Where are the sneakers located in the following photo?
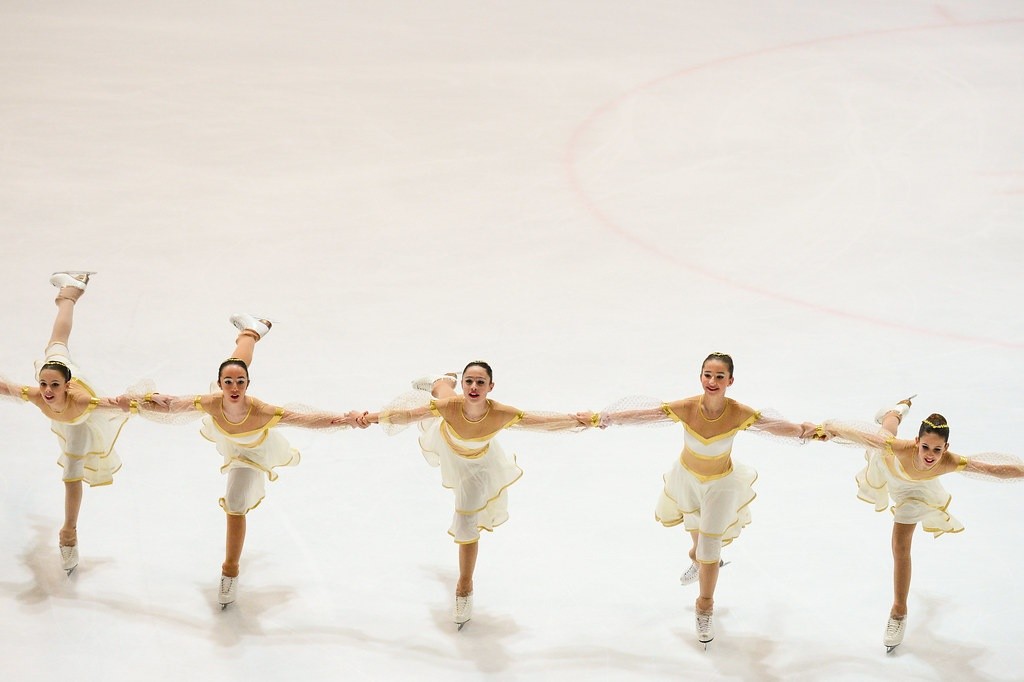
[229,312,272,345]
[453,579,473,631]
[883,605,908,653]
[695,595,716,650]
[680,548,731,585]
[49,270,97,305]
[60,526,79,576]
[412,371,462,395]
[217,563,240,611]
[875,394,917,425]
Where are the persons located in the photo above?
[1,270,1024,653]
